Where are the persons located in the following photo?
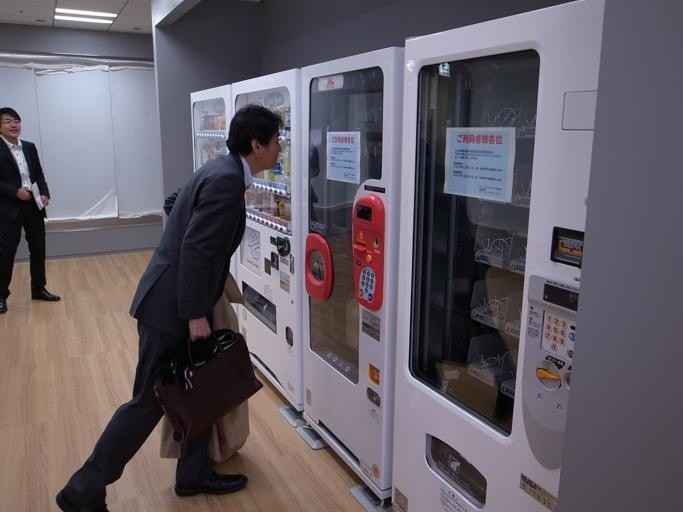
[50,103,284,511]
[0,106,61,314]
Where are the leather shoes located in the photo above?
[174,470,248,498]
[32,289,61,302]
[55,485,111,512]
[0,296,7,314]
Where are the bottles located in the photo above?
[247,103,290,220]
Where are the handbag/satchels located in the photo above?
[152,327,266,444]
